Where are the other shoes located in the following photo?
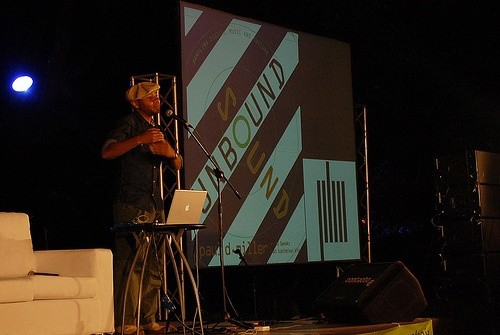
[142,323,163,331]
[115,324,137,335]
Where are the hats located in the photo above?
[125,81,160,100]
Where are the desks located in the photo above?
[109,223,208,335]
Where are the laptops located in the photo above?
[156,189,207,225]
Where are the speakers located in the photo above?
[317,261,428,326]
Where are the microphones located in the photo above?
[166,110,192,128]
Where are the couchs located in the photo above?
[0,212,115,335]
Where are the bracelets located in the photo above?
[170,153,178,159]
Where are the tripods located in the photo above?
[177,123,254,331]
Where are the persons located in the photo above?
[101,81,183,335]
